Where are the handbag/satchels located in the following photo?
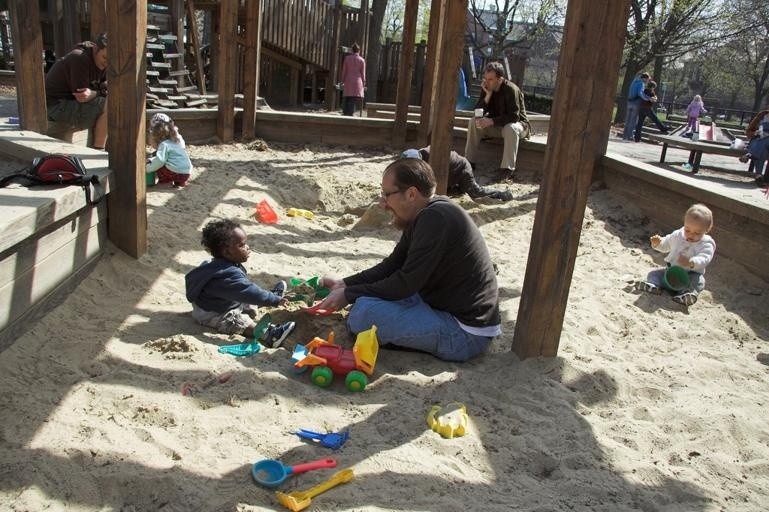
[28,153,93,186]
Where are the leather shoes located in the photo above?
[500,173,514,185]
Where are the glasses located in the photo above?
[381,189,407,199]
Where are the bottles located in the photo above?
[732,138,735,148]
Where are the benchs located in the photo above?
[648,132,755,173]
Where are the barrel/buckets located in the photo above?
[663,265,690,292]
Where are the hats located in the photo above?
[150,113,174,132]
[401,149,422,161]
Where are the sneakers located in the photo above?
[739,155,748,163]
[673,294,697,306]
[271,280,287,297]
[153,181,174,189]
[627,137,635,141]
[268,321,295,349]
[634,281,662,295]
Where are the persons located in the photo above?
[146,112,194,187]
[634,201,718,307]
[43,32,108,151]
[622,71,769,188]
[399,144,515,201]
[340,41,367,116]
[462,61,533,185]
[184,219,296,349]
[318,155,503,364]
[44,49,57,74]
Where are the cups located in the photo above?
[474,108,484,120]
